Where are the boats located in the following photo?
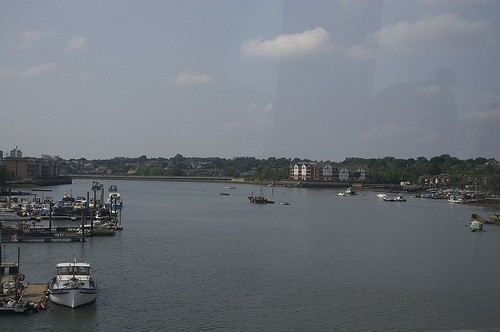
[469,213,484,232]
[279,201,290,205]
[224,186,237,190]
[447,194,463,203]
[376,193,387,198]
[0,242,26,301]
[382,193,406,203]
[337,186,358,197]
[0,181,123,220]
[46,254,98,309]
[220,192,230,196]
[248,185,276,204]
